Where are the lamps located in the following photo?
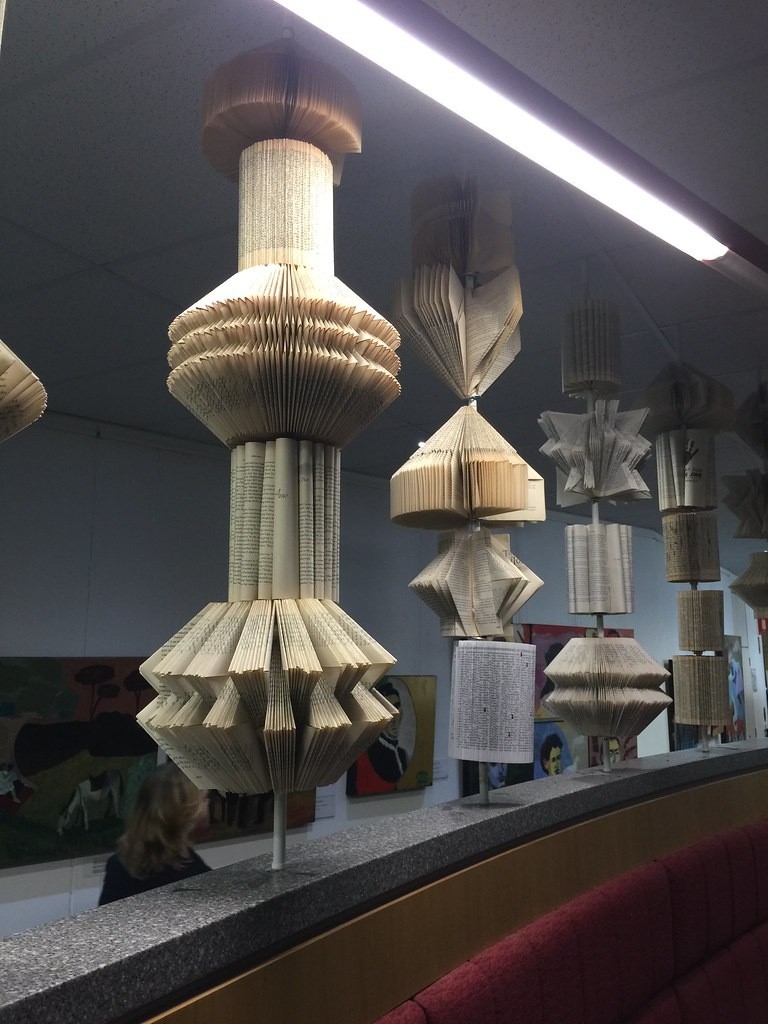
[275,1,768,299]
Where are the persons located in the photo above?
[98,759,214,906]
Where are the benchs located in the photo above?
[376,818,767,1024]
